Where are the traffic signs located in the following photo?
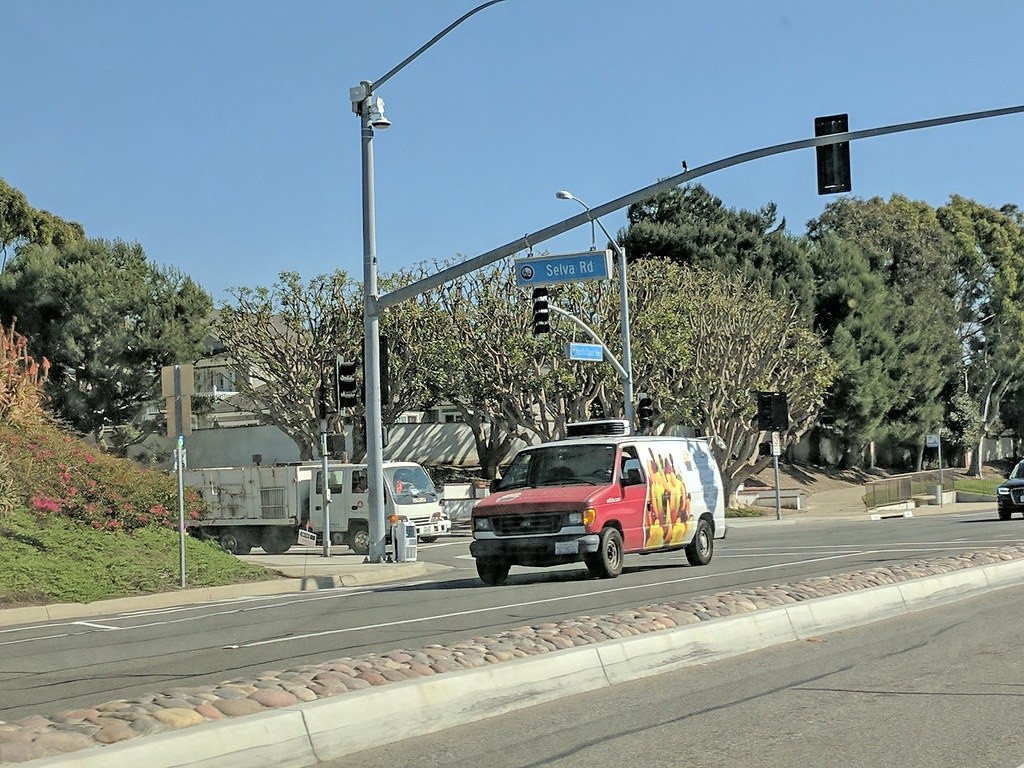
[514,250,612,287]
[564,342,603,362]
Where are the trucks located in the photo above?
[172,461,451,557]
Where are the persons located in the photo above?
[355,477,366,492]
[605,451,628,480]
[394,472,414,493]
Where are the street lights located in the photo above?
[554,191,634,435]
[360,97,393,559]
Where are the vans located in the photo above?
[471,420,726,586]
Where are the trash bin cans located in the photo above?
[392,521,417,562]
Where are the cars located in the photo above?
[997,460,1024,520]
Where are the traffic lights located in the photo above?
[757,392,774,431]
[774,392,789,431]
[335,356,357,415]
[530,286,550,335]
